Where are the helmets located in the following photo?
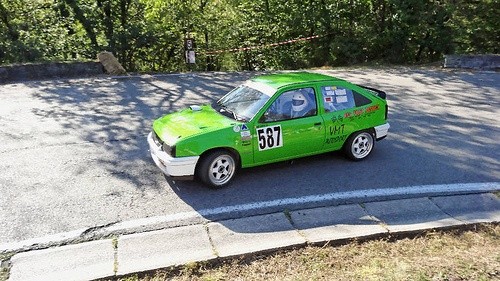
[292,91,310,111]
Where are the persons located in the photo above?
[286,93,313,122]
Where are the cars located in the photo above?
[147,71,391,188]
